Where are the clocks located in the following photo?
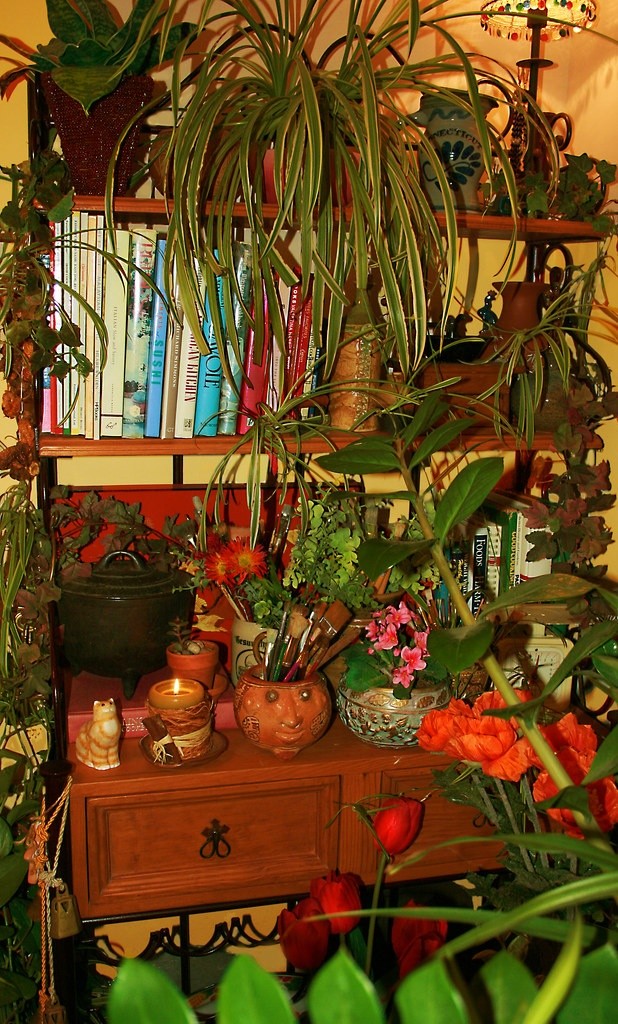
[494,638,573,714]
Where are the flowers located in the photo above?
[338,602,431,701]
[107,690,618,1024]
[48,476,448,630]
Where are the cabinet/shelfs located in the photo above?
[575,485,613,488]
[26,68,617,1024]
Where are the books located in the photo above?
[41,208,325,438]
[417,493,572,692]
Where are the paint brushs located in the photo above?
[263,599,352,683]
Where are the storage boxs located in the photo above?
[422,364,515,425]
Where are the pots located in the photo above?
[59,550,198,699]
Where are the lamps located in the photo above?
[479,0,597,181]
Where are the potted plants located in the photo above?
[164,615,219,688]
[104,0,561,383]
[0,0,205,198]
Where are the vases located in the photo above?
[233,616,281,688]
[149,127,262,200]
[338,676,453,749]
[418,95,499,214]
[327,281,388,432]
[481,282,551,362]
[511,351,584,431]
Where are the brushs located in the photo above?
[319,600,352,642]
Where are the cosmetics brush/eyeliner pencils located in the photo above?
[263,599,361,682]
